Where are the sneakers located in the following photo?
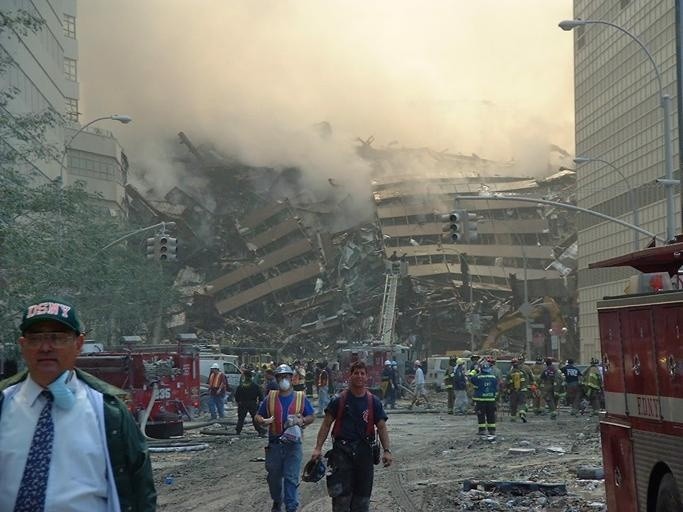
[271,500,281,512]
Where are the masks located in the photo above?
[279,379,290,391]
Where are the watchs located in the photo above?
[384,448,391,454]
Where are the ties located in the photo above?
[14,390,53,511]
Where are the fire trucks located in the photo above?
[72,343,202,437]
[337,272,408,399]
[586,240,682,512]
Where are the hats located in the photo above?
[20,297,80,335]
[415,360,422,367]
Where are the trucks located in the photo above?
[200,359,244,393]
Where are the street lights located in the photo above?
[55,114,132,259]
[559,20,683,247]
[575,158,641,250]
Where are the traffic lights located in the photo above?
[145,234,178,265]
[441,207,483,244]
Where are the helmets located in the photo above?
[275,364,293,375]
[568,359,574,364]
[511,353,524,364]
[302,456,325,482]
[384,360,397,366]
[536,355,552,365]
[210,363,219,369]
[483,355,495,369]
[592,359,599,365]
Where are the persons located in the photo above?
[409,359,431,409]
[444,354,604,422]
[255,363,315,512]
[208,353,400,433]
[467,359,497,435]
[311,361,393,512]
[0,298,158,512]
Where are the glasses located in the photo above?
[27,332,72,346]
